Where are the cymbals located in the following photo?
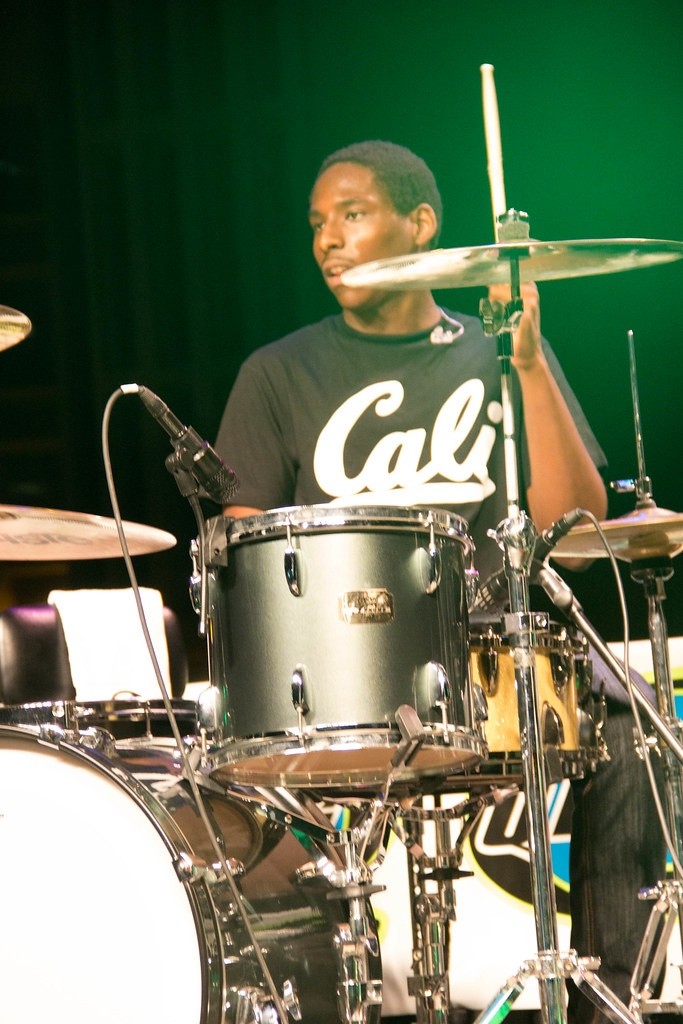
[547,501,683,562]
[0,303,33,353]
[340,237,683,289]
[0,503,179,562]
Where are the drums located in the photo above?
[467,609,606,779]
[193,504,490,790]
[0,724,389,1024]
[0,698,198,742]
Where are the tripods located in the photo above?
[474,512,683,1024]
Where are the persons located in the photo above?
[197,140,683,1023]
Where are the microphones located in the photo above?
[477,507,582,610]
[137,386,241,505]
